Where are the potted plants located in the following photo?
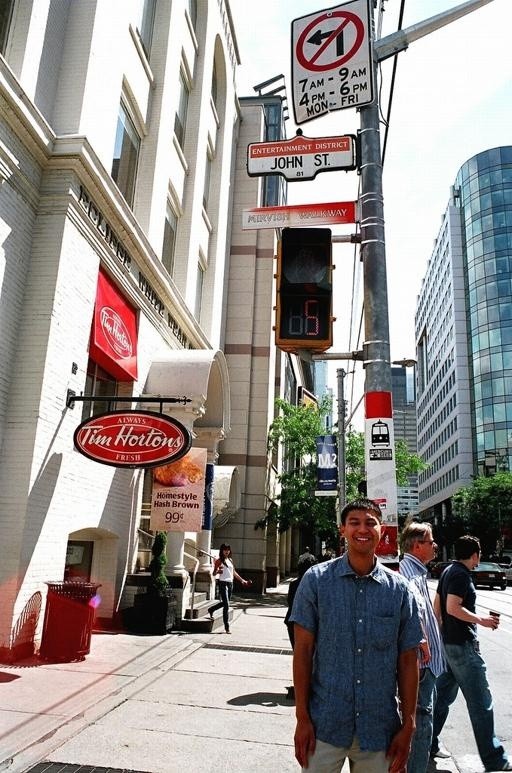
[132,531,178,635]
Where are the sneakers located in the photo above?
[208,608,214,619]
[502,755,512,770]
[226,630,231,633]
[435,749,452,758]
[286,687,295,700]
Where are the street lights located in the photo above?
[336,358,416,552]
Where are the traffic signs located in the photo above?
[246,135,354,182]
[241,200,357,230]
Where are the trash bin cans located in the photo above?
[39,581,103,663]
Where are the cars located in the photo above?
[427,560,512,590]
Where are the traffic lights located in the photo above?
[274,227,336,354]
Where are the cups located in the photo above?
[490,611,500,629]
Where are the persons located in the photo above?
[433,533,512,772]
[280,552,316,699]
[289,496,422,772]
[394,519,449,773]
[208,543,251,633]
